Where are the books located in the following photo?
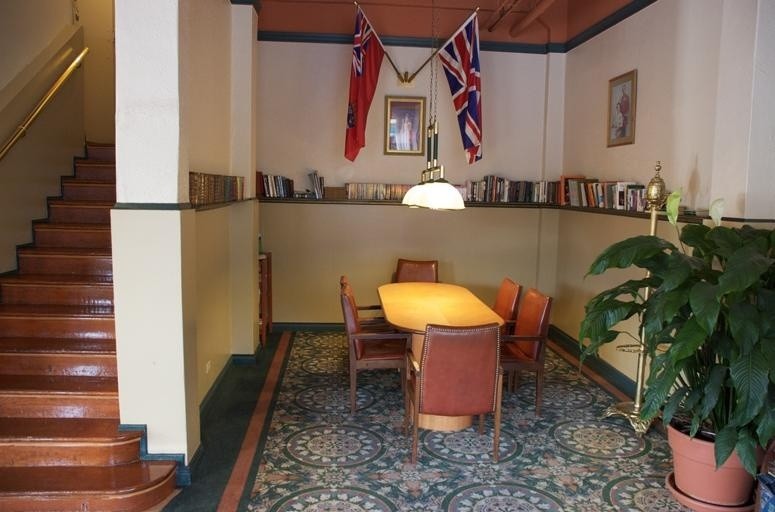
[293,192,316,199]
[561,175,667,213]
[453,176,561,205]
[678,206,696,215]
[345,183,415,200]
[256,171,294,198]
[308,172,323,199]
[320,177,324,195]
[190,172,244,207]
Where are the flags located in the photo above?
[342,7,384,163]
[437,12,483,166]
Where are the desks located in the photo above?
[376,282,504,432]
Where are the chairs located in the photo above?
[394,259,439,282]
[481,276,524,334]
[488,288,554,417]
[404,325,515,468]
[340,275,408,414]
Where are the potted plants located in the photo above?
[576,191,775,512]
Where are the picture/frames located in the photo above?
[383,95,427,156]
[607,68,637,147]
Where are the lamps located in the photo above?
[401,0,466,211]
